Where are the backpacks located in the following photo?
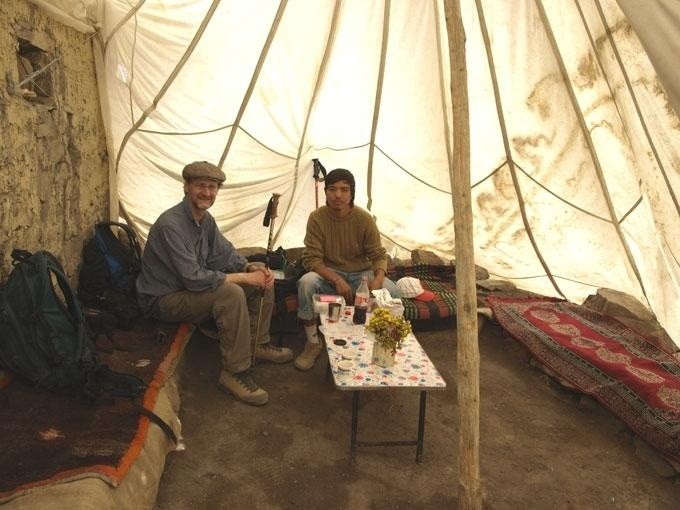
[0,248,178,448]
[77,219,143,308]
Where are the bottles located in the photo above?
[352,276,369,325]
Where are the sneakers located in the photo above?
[295,339,324,371]
[215,367,269,406]
[250,341,295,365]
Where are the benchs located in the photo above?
[267,264,480,328]
[0,311,198,509]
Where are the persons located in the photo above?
[135,161,294,405]
[294,168,402,372]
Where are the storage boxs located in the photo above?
[313,293,346,315]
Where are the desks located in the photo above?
[316,304,449,467]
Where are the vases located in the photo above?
[370,335,394,367]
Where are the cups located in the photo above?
[337,349,359,380]
[328,302,341,321]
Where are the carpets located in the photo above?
[486,296,680,460]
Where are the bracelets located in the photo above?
[333,276,342,284]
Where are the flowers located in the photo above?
[363,306,414,357]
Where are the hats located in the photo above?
[397,276,436,302]
[183,161,226,185]
